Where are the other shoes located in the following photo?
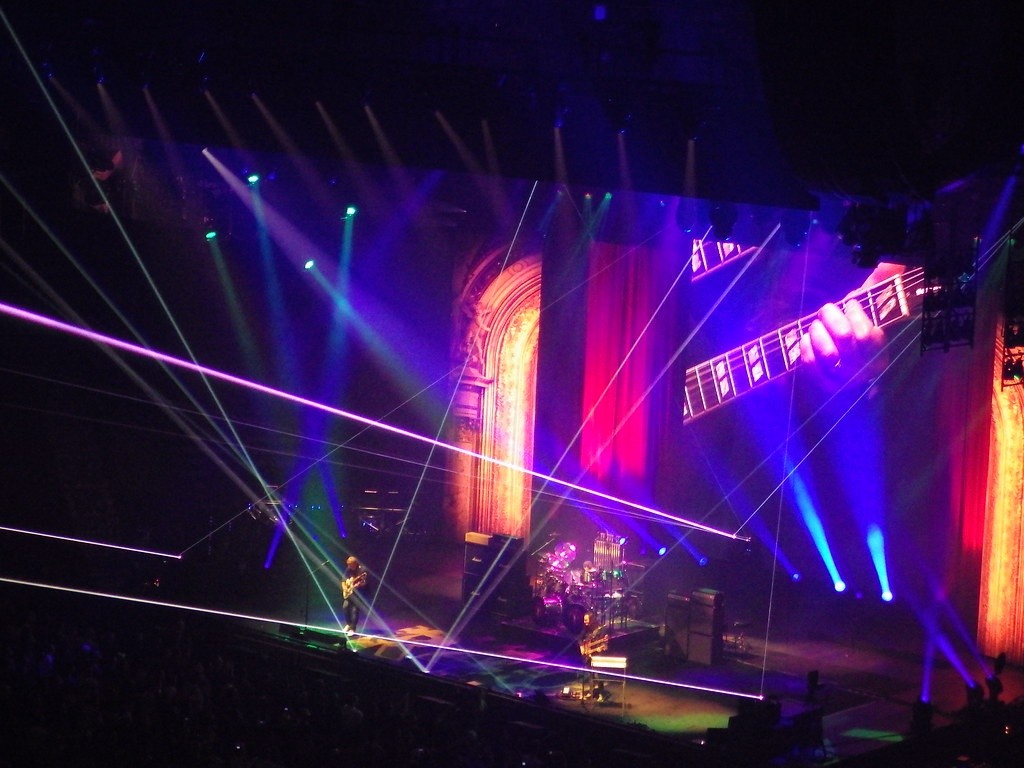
[347,630,355,637]
[342,624,351,634]
[597,690,609,702]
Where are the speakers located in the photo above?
[663,601,725,667]
[460,542,532,619]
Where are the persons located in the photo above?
[341,557,369,632]
[577,611,608,703]
[584,561,596,572]
[0,602,592,767]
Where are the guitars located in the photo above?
[340,571,368,600]
[682,261,932,432]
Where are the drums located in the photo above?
[562,600,598,635]
[532,592,564,627]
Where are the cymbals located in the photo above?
[555,542,577,563]
[546,553,569,569]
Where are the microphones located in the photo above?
[321,560,330,566]
[550,533,561,537]
